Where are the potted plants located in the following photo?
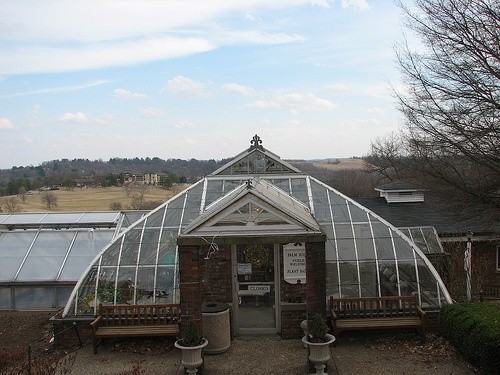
[174,317,208,369]
[302,312,337,375]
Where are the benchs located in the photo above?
[330,295,426,348]
[90,302,181,354]
